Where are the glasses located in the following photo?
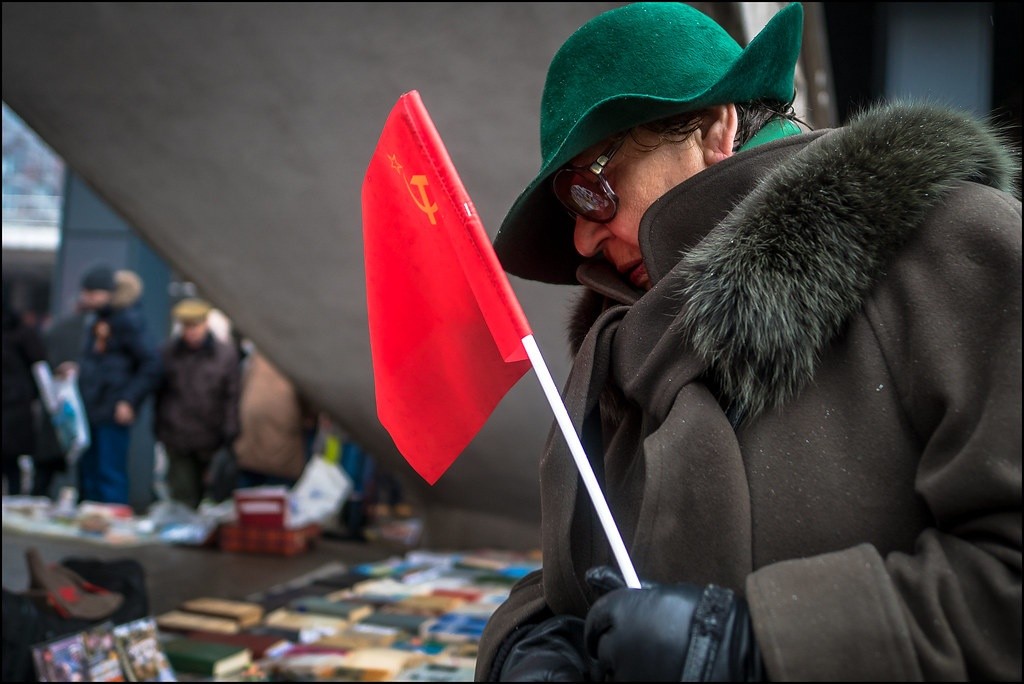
[553,111,649,221]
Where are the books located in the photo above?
[27,549,541,682]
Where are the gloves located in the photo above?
[581,566,763,684]
[501,615,587,684]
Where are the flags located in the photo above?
[358,88,539,488]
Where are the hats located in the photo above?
[175,297,207,323]
[493,1,805,286]
[83,265,117,292]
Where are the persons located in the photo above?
[0,270,53,496]
[140,295,240,504]
[240,337,307,488]
[472,1,1024,684]
[56,262,155,504]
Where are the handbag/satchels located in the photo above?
[46,369,92,465]
